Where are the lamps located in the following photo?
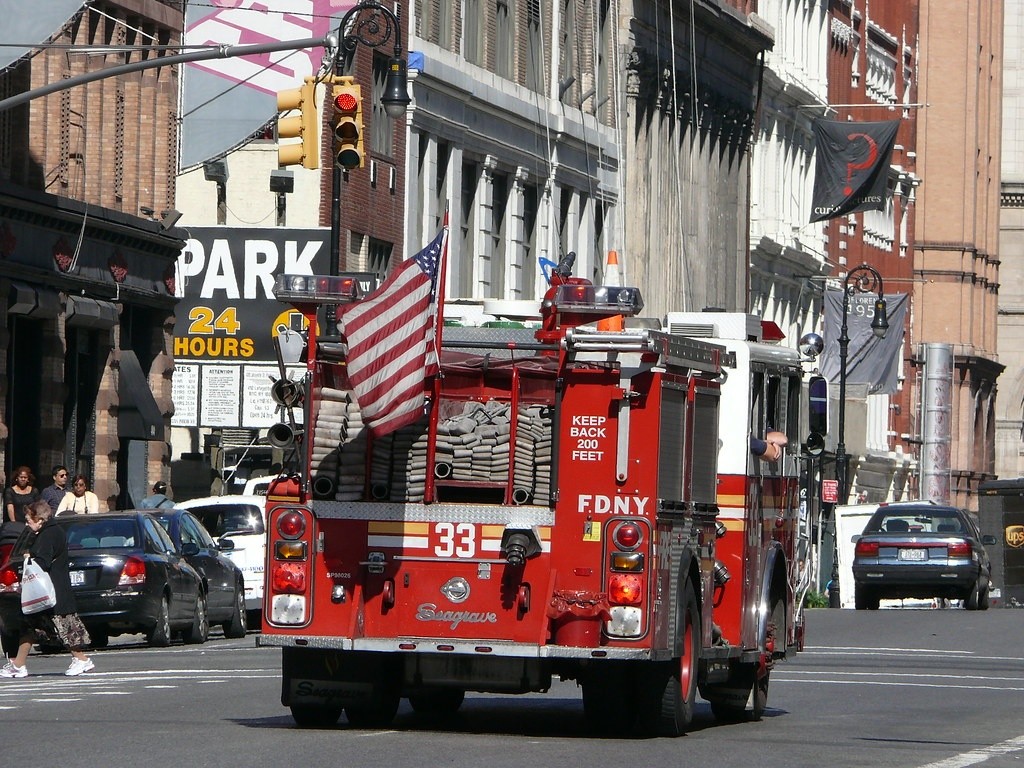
[162,208,183,231]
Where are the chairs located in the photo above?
[99,534,127,548]
[937,524,956,532]
[78,535,100,548]
[886,519,909,531]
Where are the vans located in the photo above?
[171,471,303,628]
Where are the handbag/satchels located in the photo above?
[21,555,56,613]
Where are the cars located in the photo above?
[849,502,993,611]
[7,509,248,647]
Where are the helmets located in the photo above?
[153,481,167,495]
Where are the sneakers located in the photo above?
[1,658,29,678]
[64,656,95,676]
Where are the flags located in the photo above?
[339,225,447,437]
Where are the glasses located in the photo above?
[57,472,69,478]
[73,481,86,487]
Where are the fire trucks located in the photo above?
[251,271,816,738]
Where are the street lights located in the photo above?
[833,265,890,508]
[323,1,412,335]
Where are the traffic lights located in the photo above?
[275,84,319,172]
[332,83,366,171]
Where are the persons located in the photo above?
[4,466,39,523]
[799,560,808,608]
[141,481,175,509]
[55,474,99,517]
[747,422,788,463]
[0,500,94,678]
[40,465,72,518]
